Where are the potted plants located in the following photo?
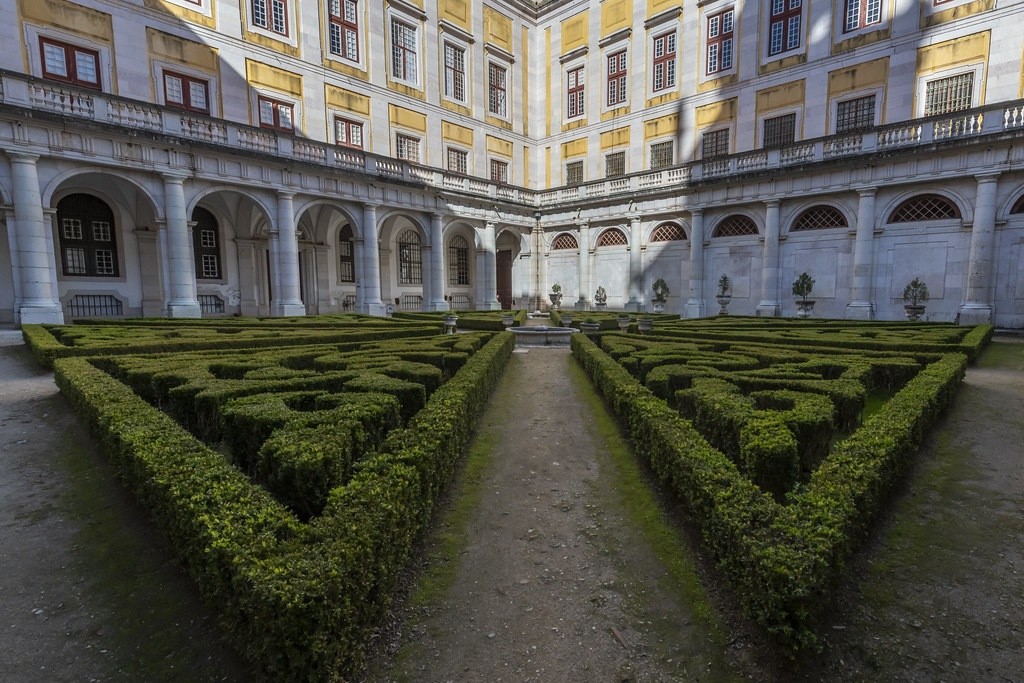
[548,283,563,311]
[650,278,669,313]
[716,274,733,315]
[791,272,819,319]
[901,277,929,320]
[594,286,608,311]
